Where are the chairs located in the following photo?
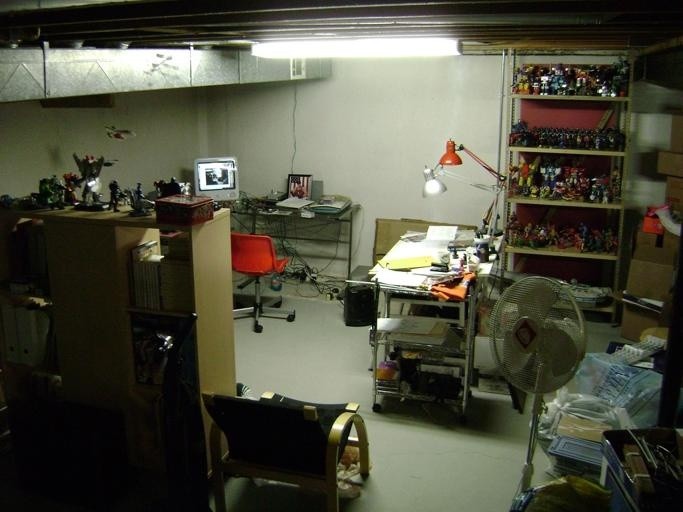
[198,388,375,511]
[228,232,297,334]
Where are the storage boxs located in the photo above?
[153,193,216,227]
[604,112,683,367]
[372,218,481,266]
[472,331,511,397]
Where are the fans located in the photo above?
[487,275,589,512]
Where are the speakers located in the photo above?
[344,286,374,326]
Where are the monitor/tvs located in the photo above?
[194,156,240,201]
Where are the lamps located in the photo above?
[421,164,502,262]
[247,36,468,62]
[438,134,508,238]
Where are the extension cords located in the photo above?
[285,273,318,284]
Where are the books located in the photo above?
[128,240,165,312]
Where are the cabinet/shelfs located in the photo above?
[501,48,634,327]
[598,425,682,511]
[0,203,235,511]
[371,233,505,414]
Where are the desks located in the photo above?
[213,198,362,302]
